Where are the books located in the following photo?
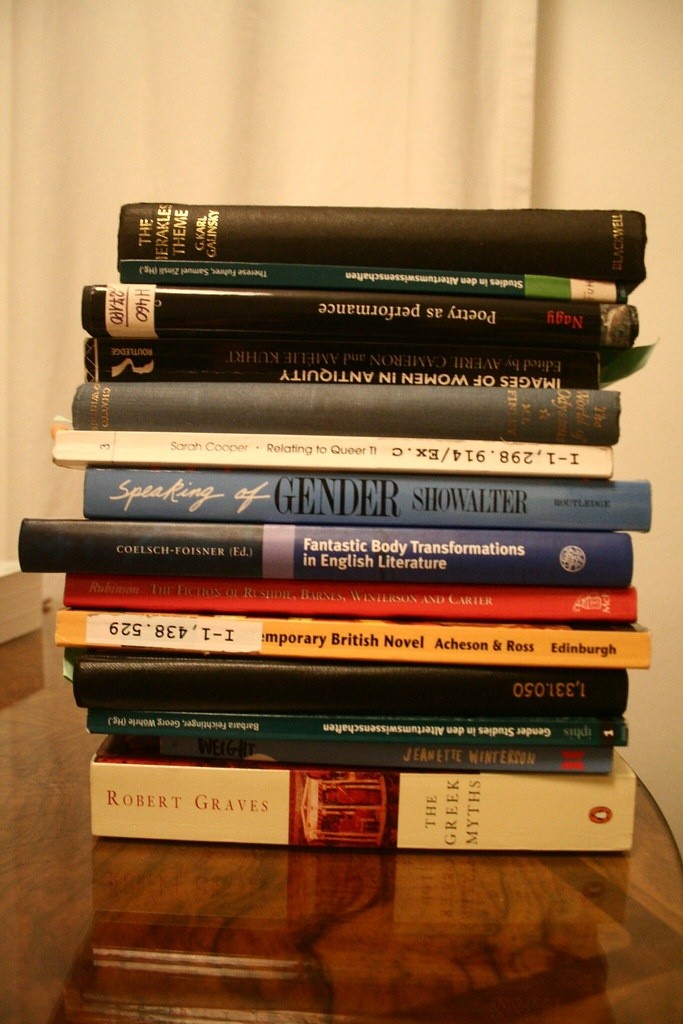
[18,200,652,851]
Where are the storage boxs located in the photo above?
[0,562,45,710]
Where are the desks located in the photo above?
[1,595,682,1024]
